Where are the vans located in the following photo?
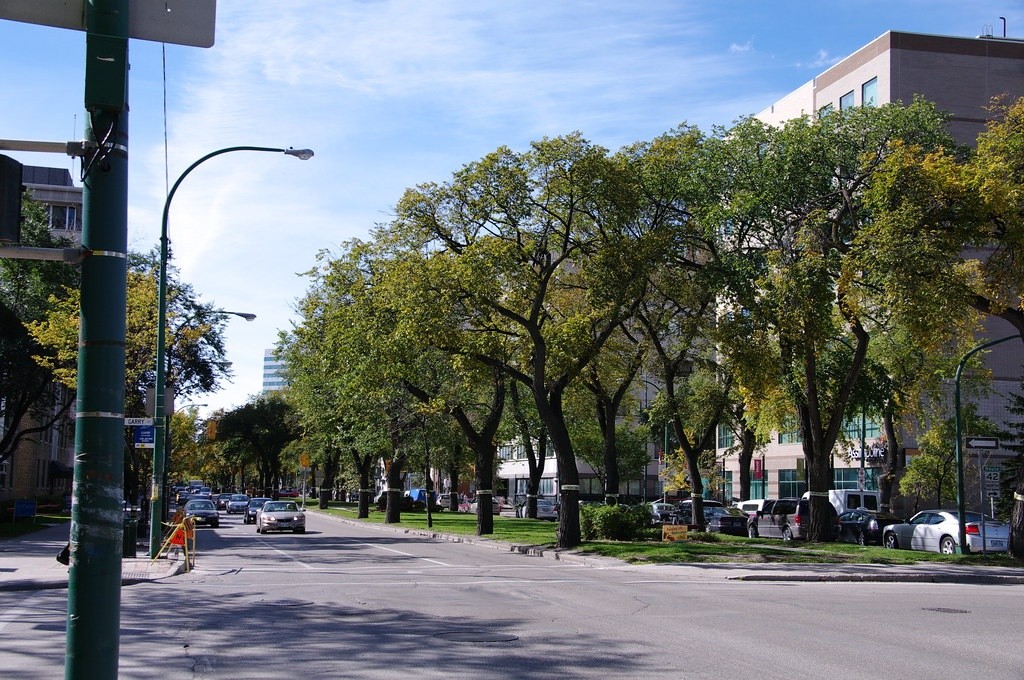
[408,488,436,505]
[803,489,882,519]
[748,496,808,540]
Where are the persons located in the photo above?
[285,504,293,510]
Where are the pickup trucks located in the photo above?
[730,499,777,518]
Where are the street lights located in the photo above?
[617,379,668,524]
[151,146,313,559]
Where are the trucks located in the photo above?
[189,480,203,492]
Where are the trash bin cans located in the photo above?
[132,515,146,538]
[123,518,137,558]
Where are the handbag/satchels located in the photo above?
[56,543,70,565]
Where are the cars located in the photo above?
[244,497,275,524]
[307,487,359,503]
[514,499,558,521]
[459,497,500,515]
[437,495,450,508]
[279,487,298,498]
[882,508,1011,555]
[640,498,748,536]
[837,509,903,545]
[184,499,220,528]
[226,494,250,514]
[174,487,233,509]
[256,501,307,534]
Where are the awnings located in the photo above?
[52,460,73,479]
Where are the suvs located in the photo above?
[373,490,388,503]
